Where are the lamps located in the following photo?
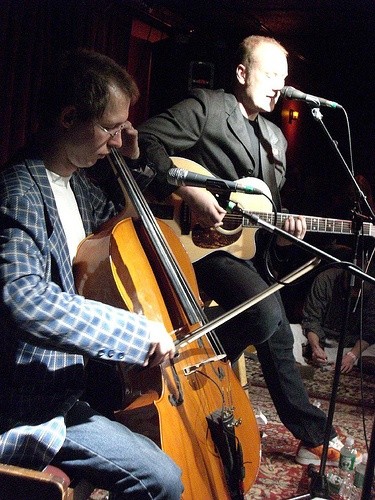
[289,109,299,124]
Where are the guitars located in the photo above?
[155,155,375,264]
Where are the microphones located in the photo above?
[167,168,263,196]
[281,86,343,109]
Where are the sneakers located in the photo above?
[295,435,362,467]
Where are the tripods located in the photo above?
[208,189,375,500]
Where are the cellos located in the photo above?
[70,143,263,500]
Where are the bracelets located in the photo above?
[347,352,356,361]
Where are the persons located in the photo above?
[0,50,182,500]
[331,174,375,250]
[300,260,375,374]
[135,34,363,466]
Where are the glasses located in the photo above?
[74,109,126,138]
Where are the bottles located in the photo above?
[337,436,357,499]
[350,452,368,500]
